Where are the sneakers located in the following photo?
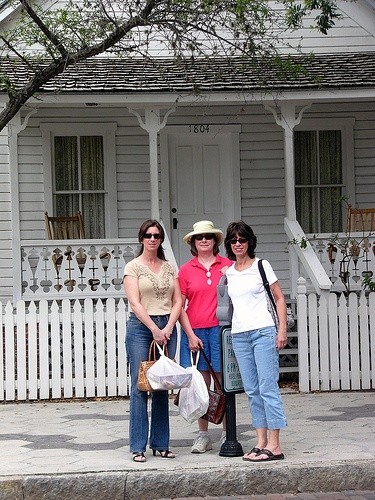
[191,433,226,453]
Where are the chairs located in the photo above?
[328,205,375,265]
[45,212,108,285]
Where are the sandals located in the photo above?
[133,448,175,462]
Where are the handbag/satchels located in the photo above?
[258,258,296,331]
[137,339,228,424]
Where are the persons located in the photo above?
[224,220,289,462]
[123,218,182,462]
[172,220,236,454]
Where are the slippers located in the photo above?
[242,446,284,462]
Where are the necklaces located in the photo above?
[199,256,212,268]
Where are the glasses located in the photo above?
[142,234,162,239]
[229,237,249,243]
[194,234,216,240]
[205,271,213,286]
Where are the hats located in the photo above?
[183,220,225,246]
